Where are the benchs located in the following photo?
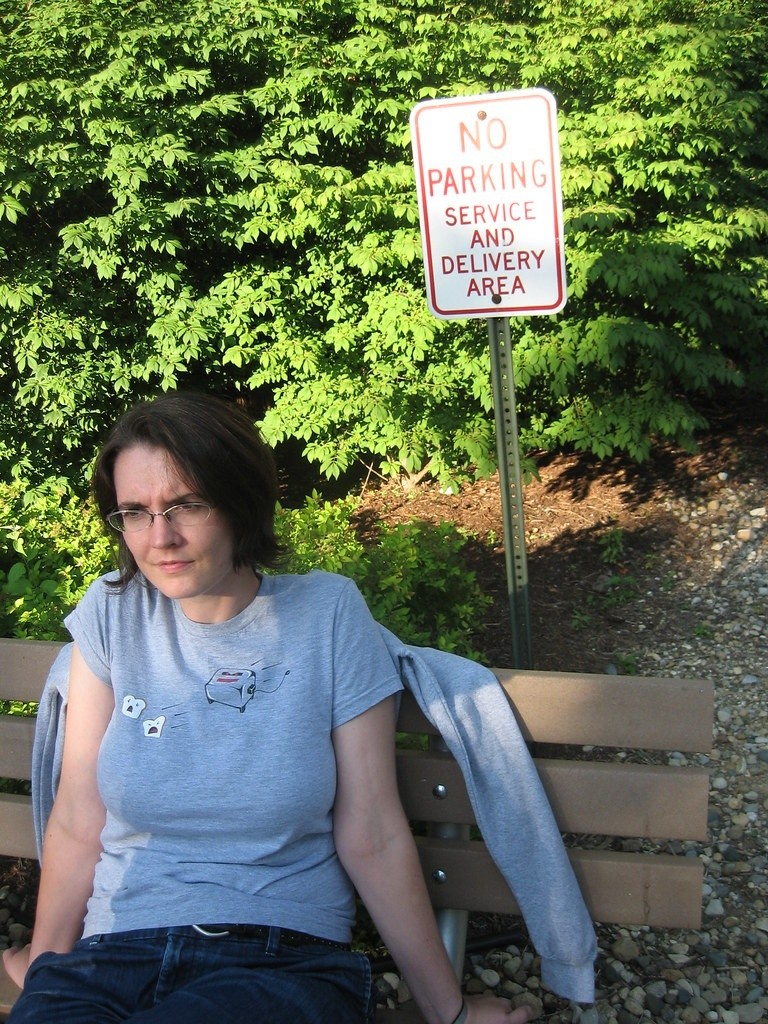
[0,636,715,1021]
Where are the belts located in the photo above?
[192,917,351,952]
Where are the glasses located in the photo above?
[105,502,214,535]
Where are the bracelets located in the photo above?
[450,996,467,1024]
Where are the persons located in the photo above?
[0,389,532,1024]
